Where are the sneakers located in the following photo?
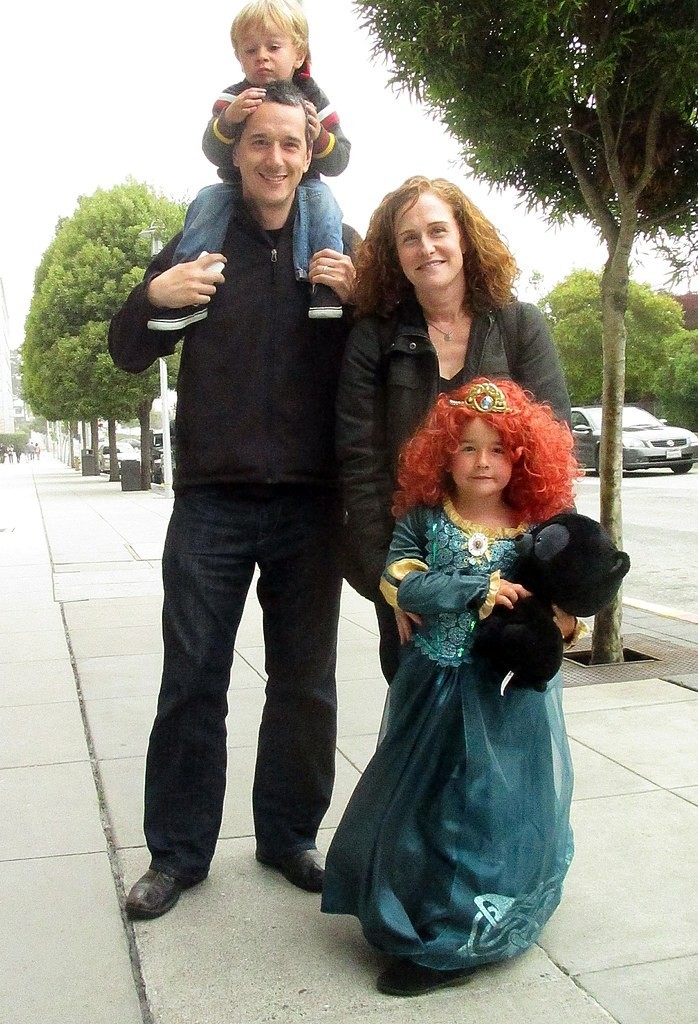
[377,955,477,996]
[308,283,342,319]
[147,305,208,332]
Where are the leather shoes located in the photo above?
[125,867,188,917]
[256,843,326,892]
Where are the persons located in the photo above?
[0,442,41,464]
[320,378,593,997]
[108,77,363,916]
[332,175,571,685]
[148,0,351,331]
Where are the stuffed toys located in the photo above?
[479,514,630,697]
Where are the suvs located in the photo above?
[147,430,177,484]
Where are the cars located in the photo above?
[96,440,140,474]
[569,403,698,476]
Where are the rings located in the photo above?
[323,265,327,274]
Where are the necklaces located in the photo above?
[426,314,467,341]
